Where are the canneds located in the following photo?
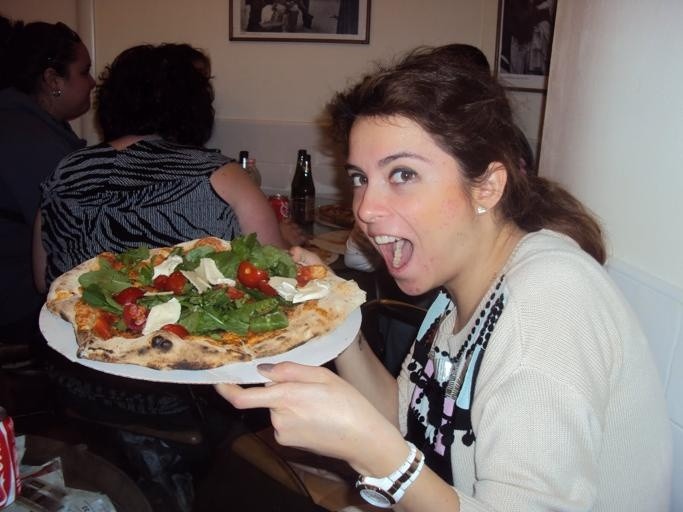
[0,407,23,510]
[269,194,291,222]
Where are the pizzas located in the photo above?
[45,232,369,373]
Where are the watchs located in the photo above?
[352,440,427,510]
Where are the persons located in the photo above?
[0,17,95,333]
[210,38,676,512]
[30,41,285,428]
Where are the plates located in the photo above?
[320,253,339,265]
[313,230,348,256]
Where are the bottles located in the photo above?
[238,150,248,171]
[245,158,260,193]
[290,148,314,228]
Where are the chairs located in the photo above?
[185,298,435,512]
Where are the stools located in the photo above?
[65,405,214,445]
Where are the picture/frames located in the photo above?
[228,0,369,43]
[493,0,556,92]
